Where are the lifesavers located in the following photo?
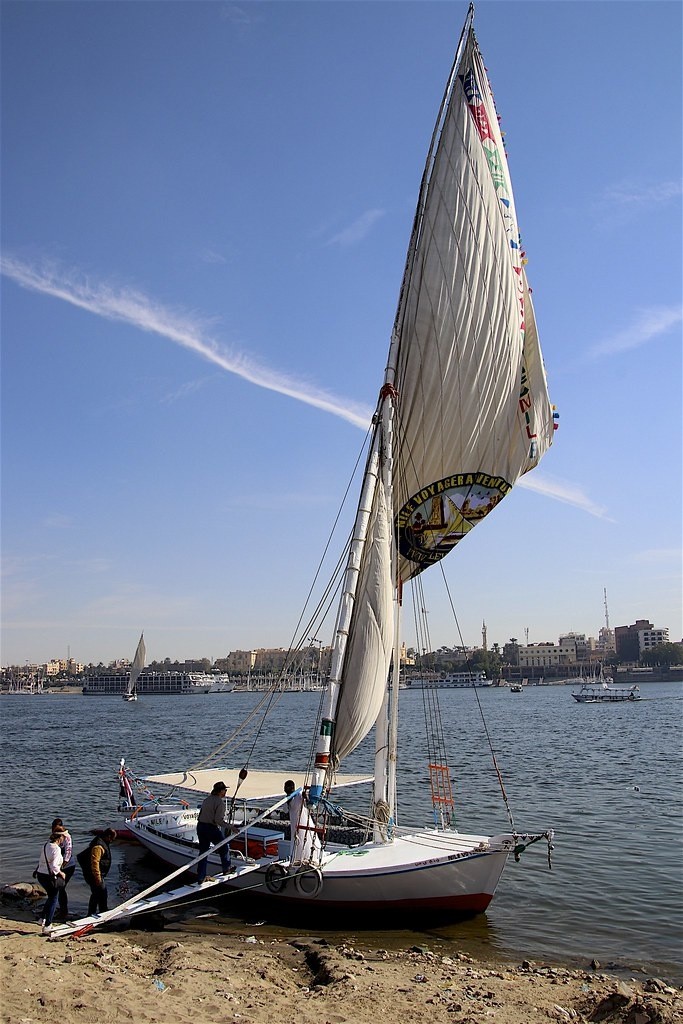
[294,864,323,899]
[264,863,288,894]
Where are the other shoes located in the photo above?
[199,875,211,884]
[87,912,101,919]
[223,865,237,875]
[38,918,46,924]
[43,924,57,932]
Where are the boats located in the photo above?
[511,686,523,692]
[82,670,236,694]
[407,670,493,688]
[570,685,641,703]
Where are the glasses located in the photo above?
[224,789,227,791]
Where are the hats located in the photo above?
[214,782,230,792]
[54,829,70,837]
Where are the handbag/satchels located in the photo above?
[50,874,66,887]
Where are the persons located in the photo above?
[51,818,76,920]
[76,827,117,917]
[197,782,240,885]
[284,780,301,806]
[36,827,72,930]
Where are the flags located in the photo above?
[119,777,135,806]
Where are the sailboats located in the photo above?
[122,629,147,700]
[124,1,563,927]
